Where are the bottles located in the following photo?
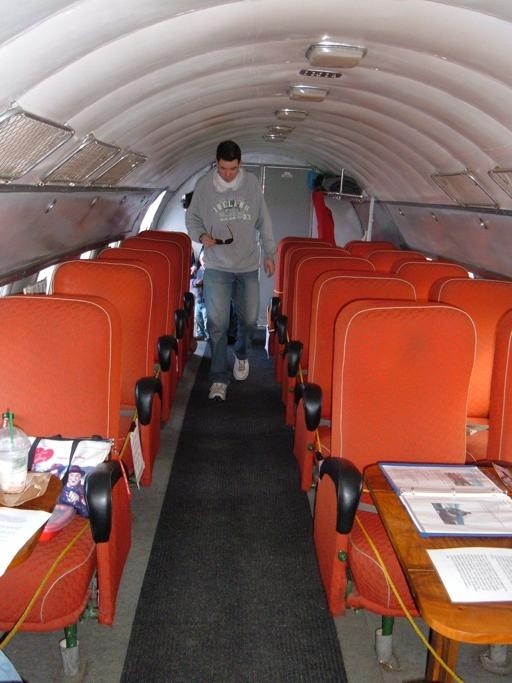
[1,407,17,432]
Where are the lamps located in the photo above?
[286,84,330,102]
[305,42,368,69]
[262,110,310,143]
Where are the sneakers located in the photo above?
[208,382,228,402]
[194,334,212,342]
[232,349,250,382]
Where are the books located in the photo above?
[378,459,512,538]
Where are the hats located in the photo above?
[44,462,66,473]
[68,465,86,477]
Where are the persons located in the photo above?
[59,464,90,518]
[184,139,277,401]
[189,249,211,342]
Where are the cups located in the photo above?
[0,426,33,495]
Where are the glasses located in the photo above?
[210,223,234,245]
